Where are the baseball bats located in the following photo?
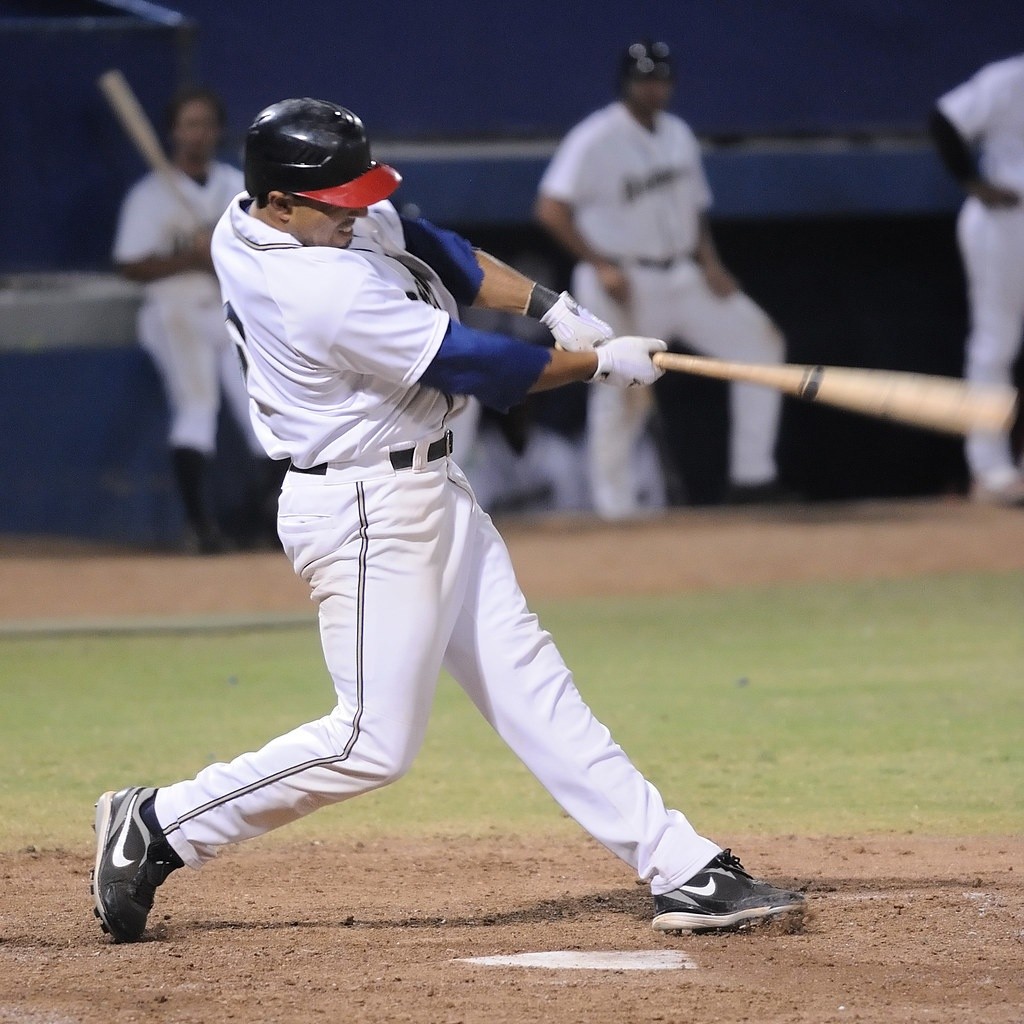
[99,68,206,231]
[552,339,1018,439]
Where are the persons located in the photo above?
[115,94,282,555]
[89,98,809,942]
[535,39,787,520]
[932,55,1024,506]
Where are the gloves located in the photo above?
[584,336,668,388]
[540,290,615,352]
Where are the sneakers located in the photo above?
[90,786,185,944]
[652,848,808,930]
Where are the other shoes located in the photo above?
[969,467,1024,504]
[203,534,229,553]
[734,480,799,505]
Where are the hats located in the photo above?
[244,97,402,208]
[622,40,673,80]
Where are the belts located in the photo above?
[289,429,454,475]
[612,254,698,271]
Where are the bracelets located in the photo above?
[525,284,557,319]
